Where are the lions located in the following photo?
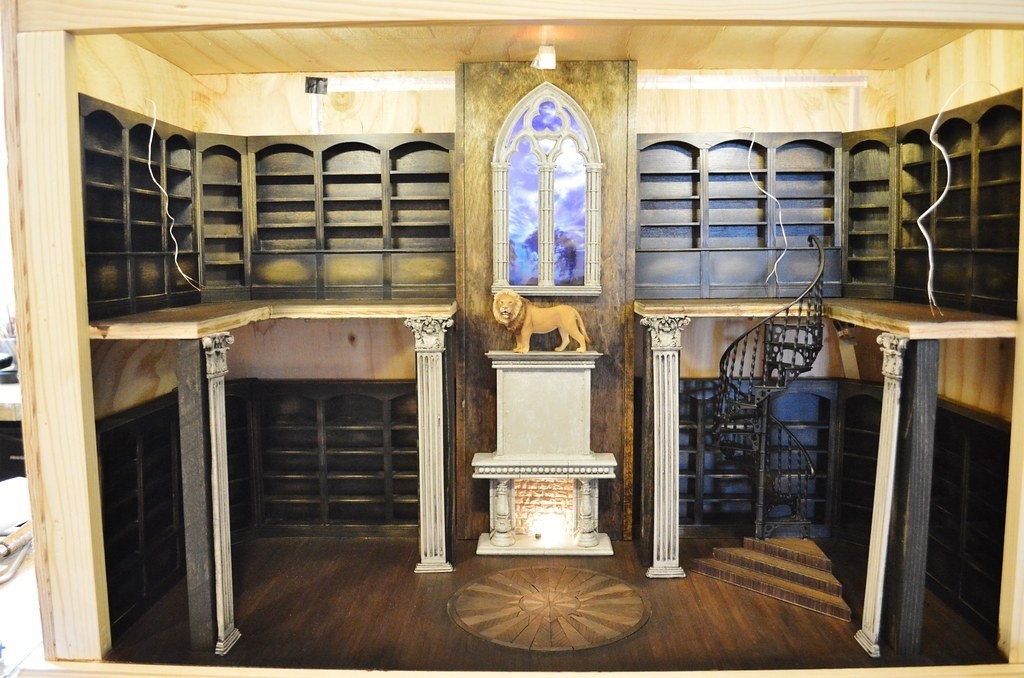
[492,289,593,352]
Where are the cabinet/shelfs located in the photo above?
[95,376,419,642]
[77,90,457,321]
[635,84,1023,318]
[678,378,1013,649]
